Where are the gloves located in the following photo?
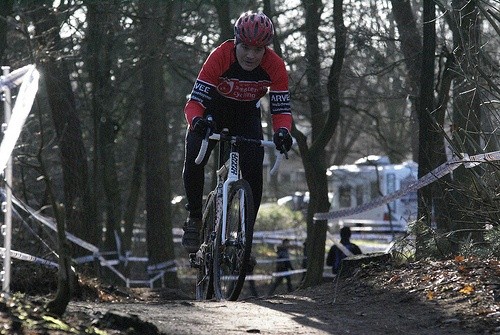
[273,128,293,154]
[192,117,217,137]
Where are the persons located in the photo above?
[182,13,293,254]
[326,226,362,275]
[267,239,296,295]
[300,243,308,281]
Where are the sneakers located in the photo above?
[182,219,202,253]
[237,255,254,273]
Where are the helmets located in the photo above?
[234,10,274,46]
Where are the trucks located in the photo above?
[326,155,417,234]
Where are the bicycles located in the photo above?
[189,114,289,301]
